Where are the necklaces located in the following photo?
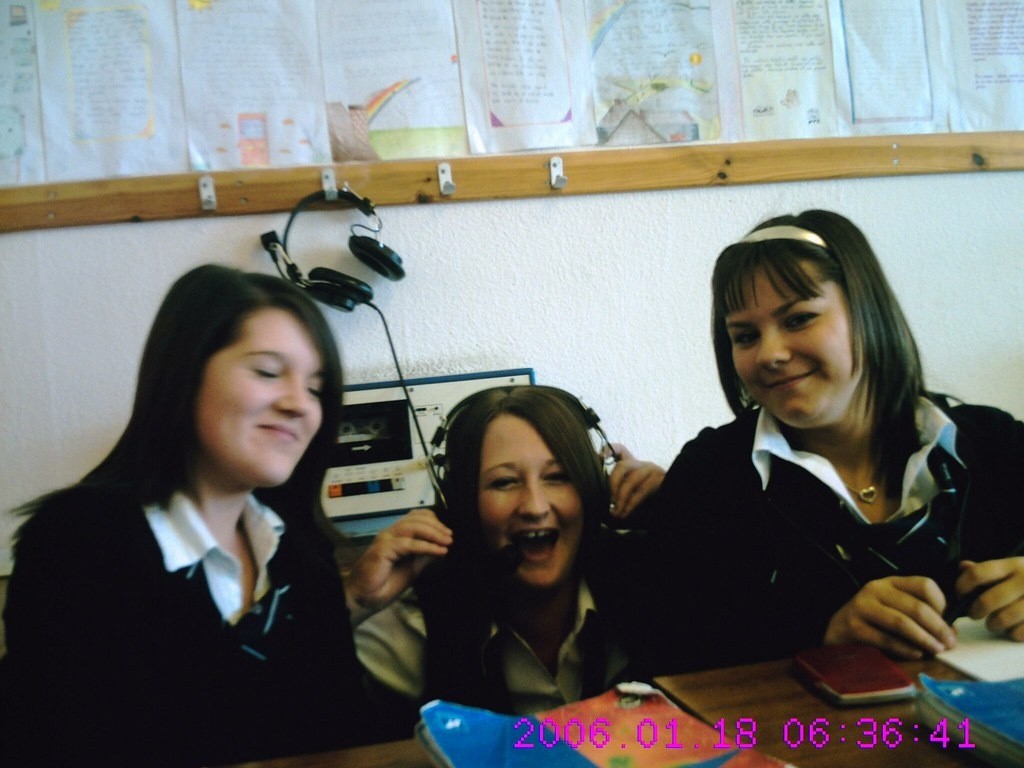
[848,485,876,503]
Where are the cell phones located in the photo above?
[795,641,918,705]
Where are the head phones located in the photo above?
[262,181,406,312]
[428,383,626,541]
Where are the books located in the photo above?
[794,614,1024,768]
[417,681,798,768]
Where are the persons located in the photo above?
[0,264,418,768]
[345,386,667,713]
[628,210,1024,659]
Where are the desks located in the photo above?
[245,611,1024,768]
